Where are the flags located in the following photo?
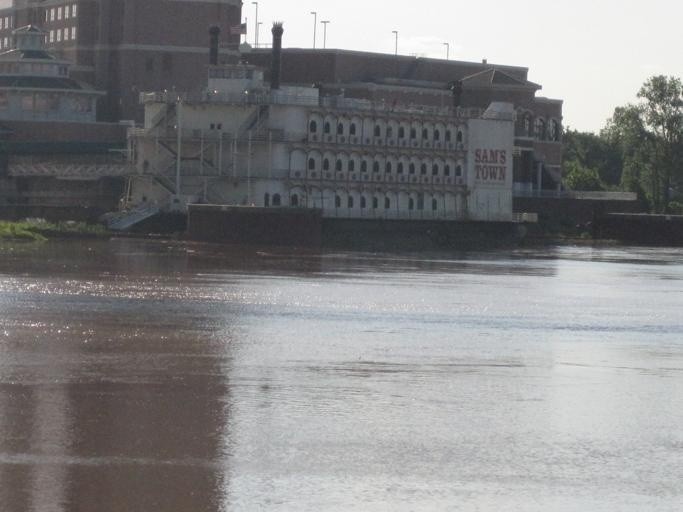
[230,23,246,35]
